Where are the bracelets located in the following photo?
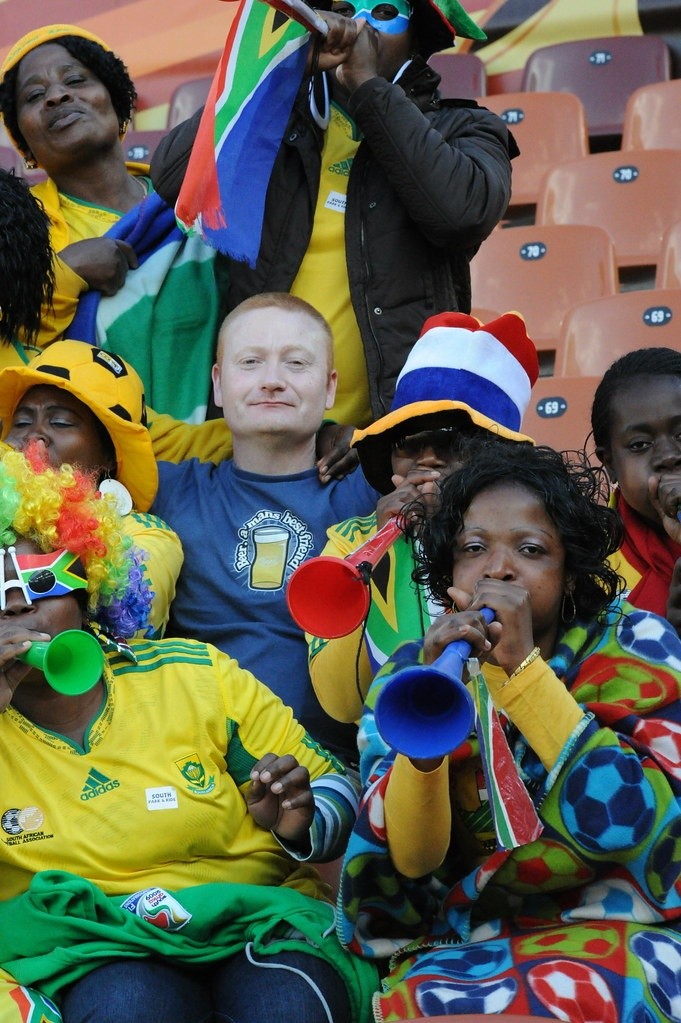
[501,647,541,688]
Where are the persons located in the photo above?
[0,0,681,1023]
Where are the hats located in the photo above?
[0,24,135,168]
[350,311,541,495]
[409,0,488,52]
[0,340,160,513]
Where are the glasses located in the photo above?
[392,423,480,462]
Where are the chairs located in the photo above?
[0,0,681,506]
